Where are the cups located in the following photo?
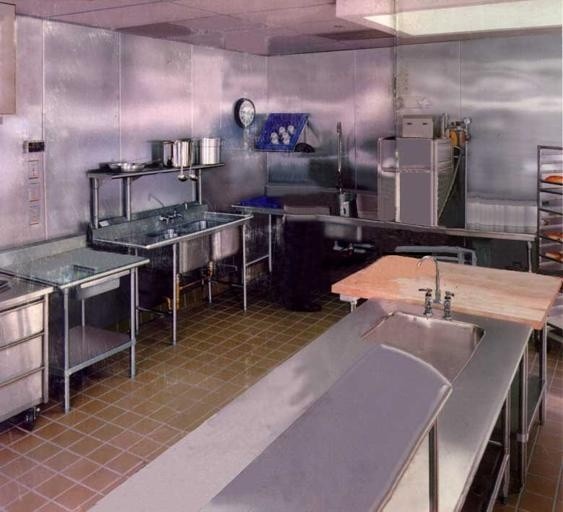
[269,125,295,145]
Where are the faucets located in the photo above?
[416,254,442,303]
[157,215,169,225]
[167,209,184,219]
[332,121,344,199]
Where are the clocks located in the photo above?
[235,98,256,129]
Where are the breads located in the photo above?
[544,249,563,261]
[546,176,563,183]
[543,231,563,242]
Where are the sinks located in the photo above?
[145,226,185,241]
[175,217,234,235]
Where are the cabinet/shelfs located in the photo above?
[535,143,562,347]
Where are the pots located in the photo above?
[197,137,220,165]
[159,138,192,168]
[102,156,161,173]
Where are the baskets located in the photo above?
[255,112,308,150]
